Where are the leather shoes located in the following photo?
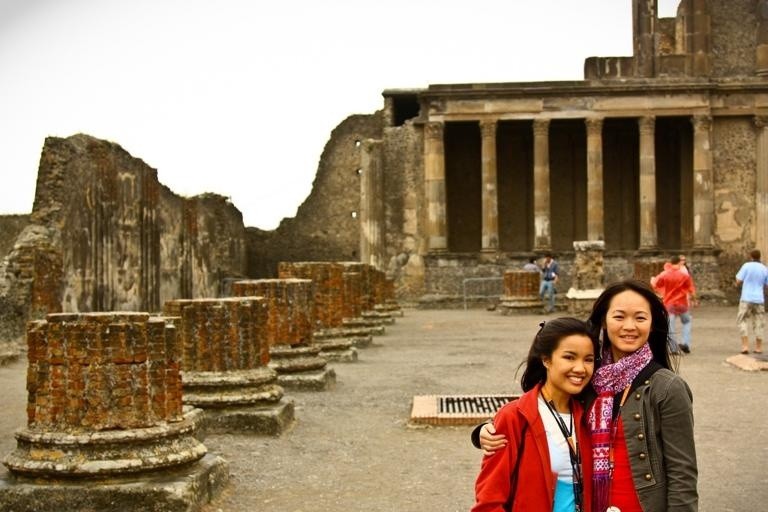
[679,344,690,355]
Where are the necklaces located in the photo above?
[540,389,585,512]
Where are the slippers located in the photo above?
[741,350,750,354]
[754,351,762,353]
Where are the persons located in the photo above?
[469,314,592,512]
[679,252,689,279]
[524,257,540,272]
[649,255,697,358]
[734,247,768,355]
[539,252,560,311]
[471,277,700,512]
